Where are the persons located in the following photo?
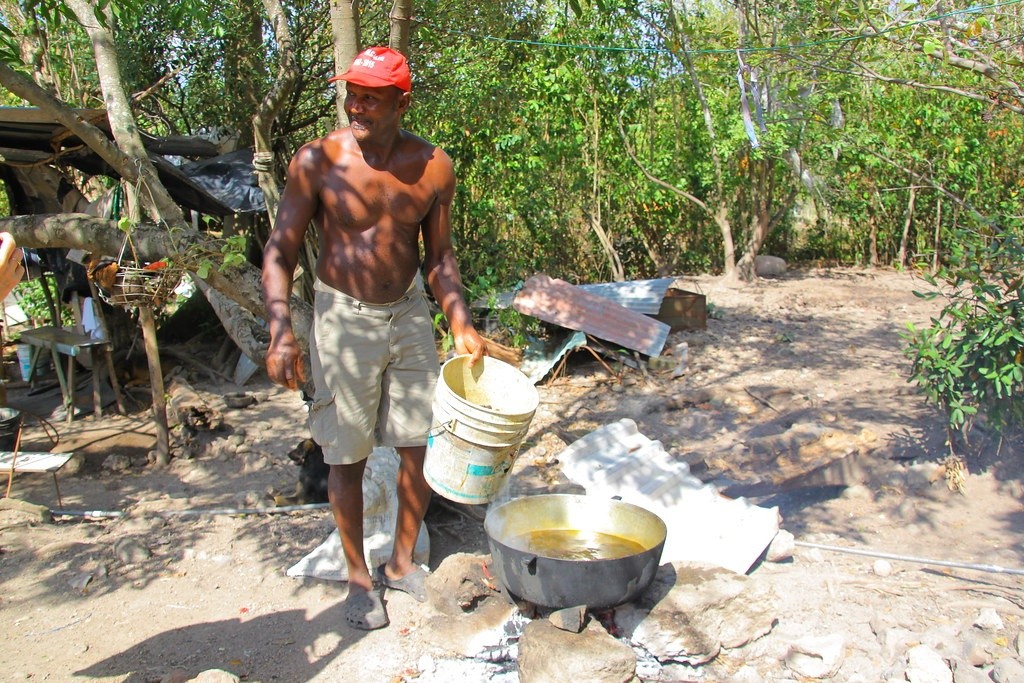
[262,48,486,630]
[0,230,26,304]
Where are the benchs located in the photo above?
[19,325,127,423]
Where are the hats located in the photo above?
[327,48,411,92]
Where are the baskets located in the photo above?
[94,266,185,308]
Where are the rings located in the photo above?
[11,257,20,264]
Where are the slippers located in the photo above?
[376,562,433,602]
[345,579,388,630]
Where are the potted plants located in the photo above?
[123,213,246,305]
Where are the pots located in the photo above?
[484,493,668,611]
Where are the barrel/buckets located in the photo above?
[420,354,540,506]
[16,343,49,382]
[0,408,20,452]
